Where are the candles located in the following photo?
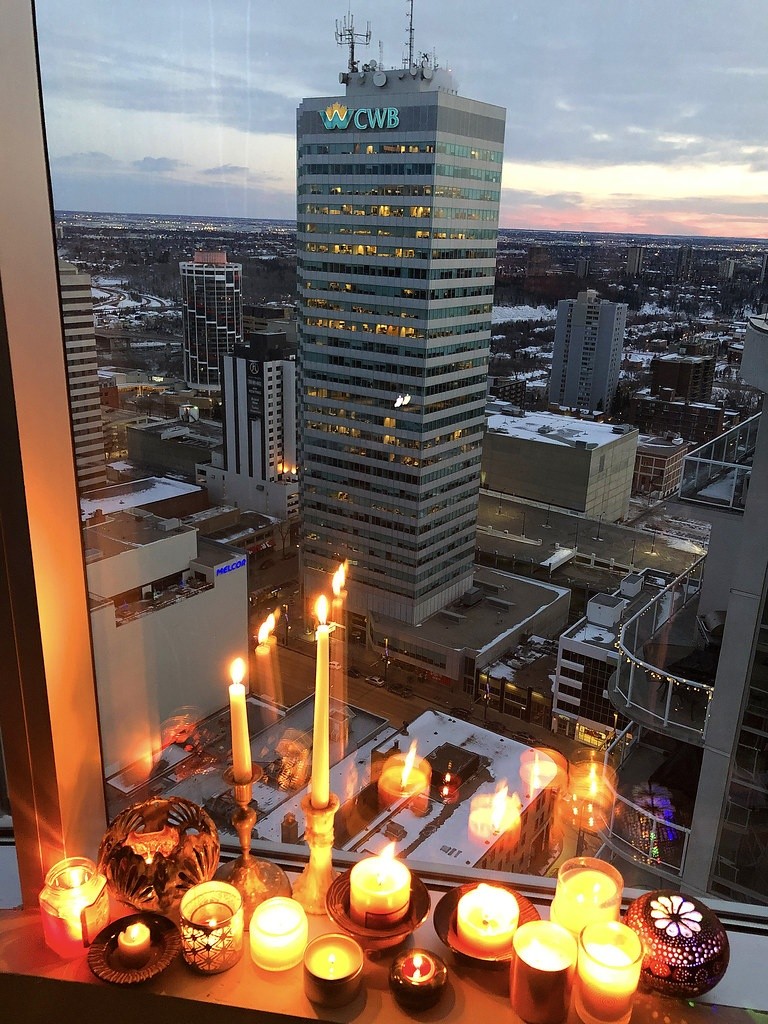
[388,949,448,1012]
[348,839,413,928]
[226,657,253,787]
[256,605,288,727]
[377,738,432,820]
[457,881,520,956]
[178,881,244,977]
[303,932,366,1011]
[511,856,644,1024]
[248,896,308,973]
[37,857,111,962]
[312,591,329,808]
[469,743,619,854]
[116,922,152,972]
[331,558,354,762]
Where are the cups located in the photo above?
[520,748,618,831]
[550,856,624,943]
[576,921,645,1024]
[511,921,576,1024]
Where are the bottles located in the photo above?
[38,857,109,960]
